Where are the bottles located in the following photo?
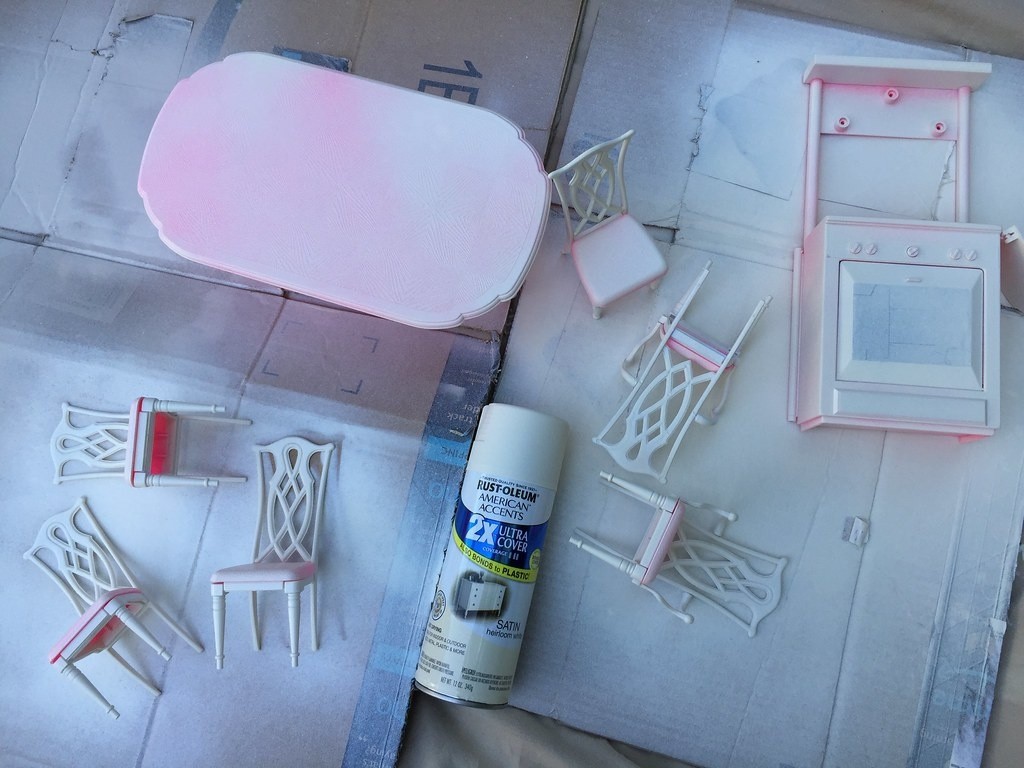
[412,402,573,708]
